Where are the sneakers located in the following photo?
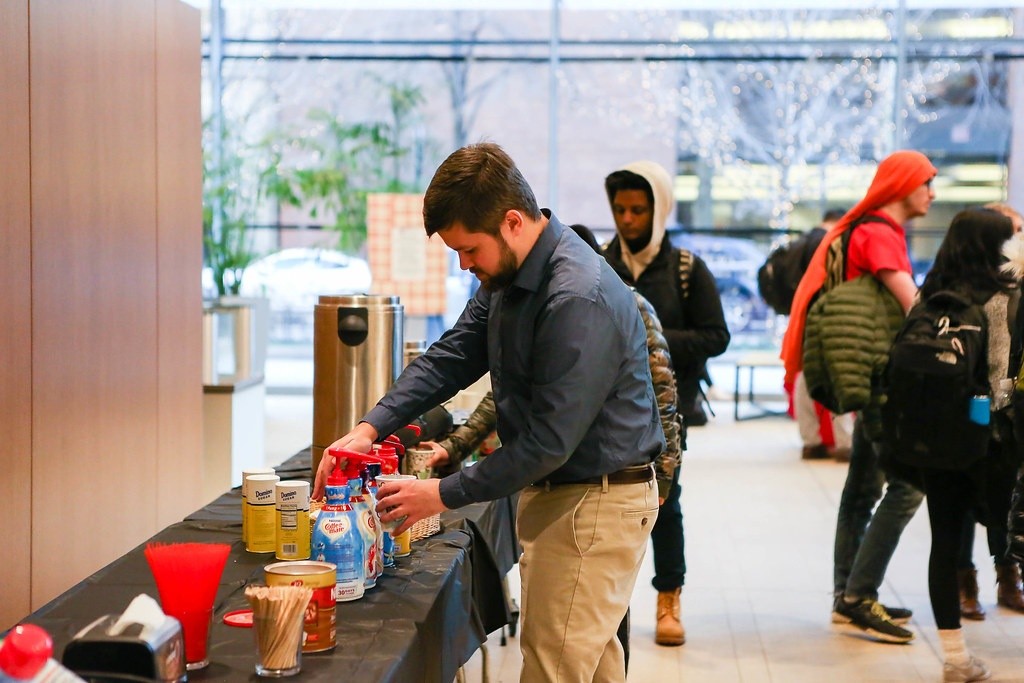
[943,655,991,683]
[834,591,914,642]
[833,594,912,624]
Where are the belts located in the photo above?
[527,465,655,484]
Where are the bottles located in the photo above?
[971,395,990,426]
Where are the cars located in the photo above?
[236,246,375,312]
[670,234,774,334]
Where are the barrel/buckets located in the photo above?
[263,560,337,654]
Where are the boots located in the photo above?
[994,559,1024,612]
[655,583,686,645]
[958,568,985,619]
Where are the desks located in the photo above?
[713,352,788,423]
[1,400,524,683]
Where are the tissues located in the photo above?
[62,593,187,683]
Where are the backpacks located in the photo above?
[758,231,825,315]
[819,210,892,293]
[875,266,1009,494]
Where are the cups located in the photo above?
[144,544,231,669]
[405,447,433,481]
[253,615,303,677]
[376,475,415,536]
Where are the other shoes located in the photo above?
[833,446,851,464]
[803,442,832,458]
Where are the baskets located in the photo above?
[409,513,441,544]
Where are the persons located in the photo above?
[787,150,938,644]
[310,143,666,683]
[756,208,858,463]
[420,224,681,683]
[603,161,731,646]
[878,204,1024,683]
